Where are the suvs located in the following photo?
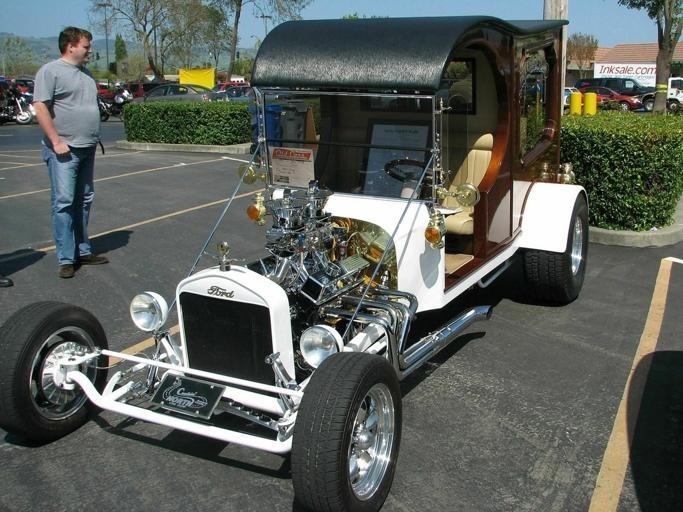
[640,77,683,111]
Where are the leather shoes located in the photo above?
[59,264,74,277]
[78,254,108,265]
[0,279,13,287]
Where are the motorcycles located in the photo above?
[98,89,135,121]
[0,89,33,125]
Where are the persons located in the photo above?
[0,275,14,288]
[33,27,109,280]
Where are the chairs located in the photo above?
[322,123,494,236]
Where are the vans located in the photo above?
[575,78,646,95]
[123,81,168,97]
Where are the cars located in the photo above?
[132,82,256,103]
[0,77,34,99]
[579,86,642,110]
[564,87,585,115]
[0,14,589,512]
[97,85,113,94]
